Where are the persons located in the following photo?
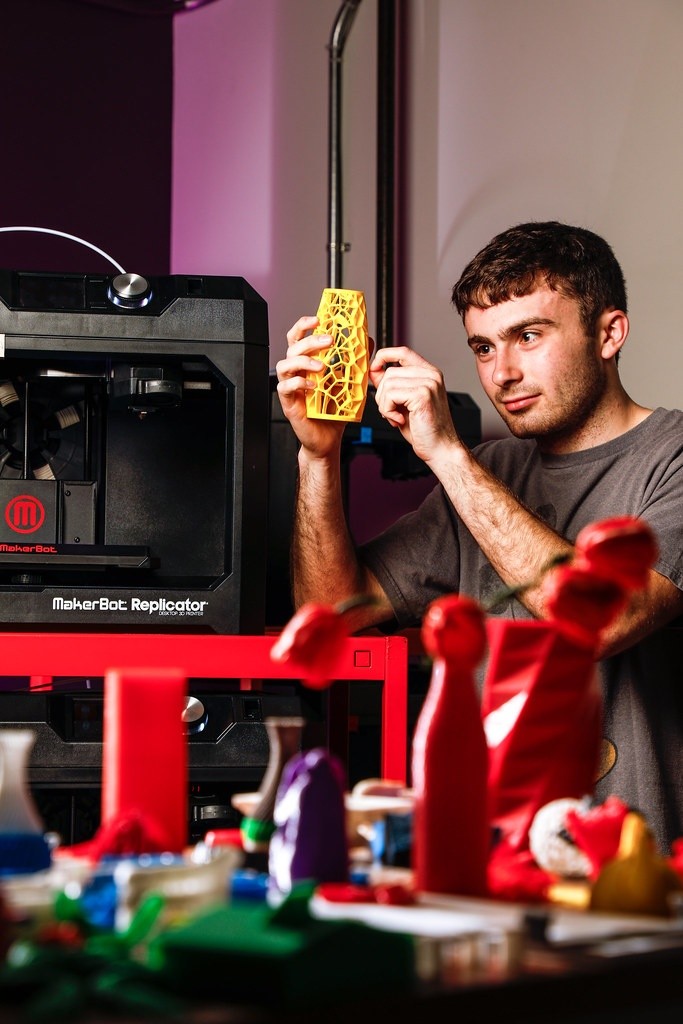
[275,224,683,854]
